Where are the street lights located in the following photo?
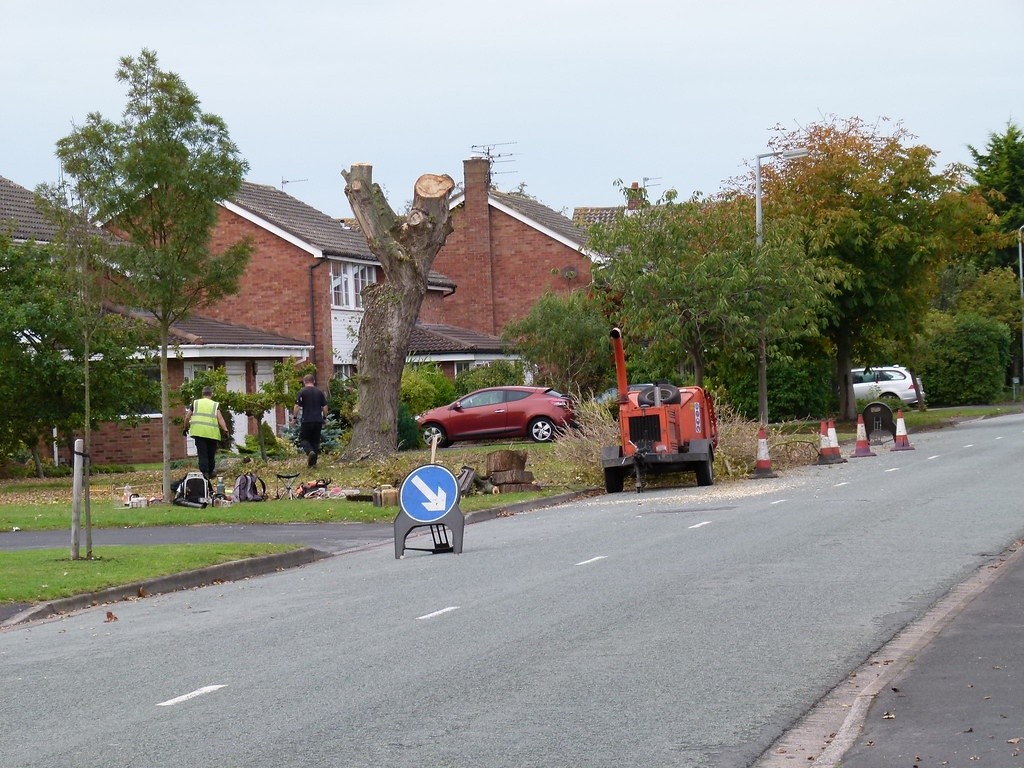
[753,146,810,424]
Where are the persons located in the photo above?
[292,374,328,468]
[182,386,228,480]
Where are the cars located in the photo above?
[414,385,578,449]
[586,380,657,422]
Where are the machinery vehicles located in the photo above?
[599,327,719,494]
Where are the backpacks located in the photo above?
[233,472,266,502]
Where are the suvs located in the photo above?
[835,363,927,411]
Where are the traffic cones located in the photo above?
[812,421,838,466]
[747,427,779,479]
[849,413,877,457]
[827,419,848,463]
[888,408,916,452]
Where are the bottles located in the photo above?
[217,482,225,495]
[124,483,131,504]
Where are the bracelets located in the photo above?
[293,417,297,419]
[323,416,326,420]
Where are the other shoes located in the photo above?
[306,452,316,468]
